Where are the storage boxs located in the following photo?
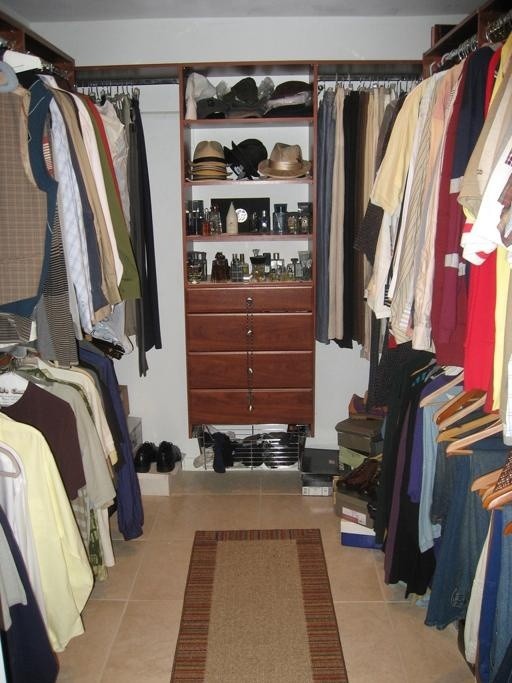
[115,384,181,499]
[332,417,389,548]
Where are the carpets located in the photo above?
[169,525,353,682]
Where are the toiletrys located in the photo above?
[186,200,312,284]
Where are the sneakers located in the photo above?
[335,456,383,502]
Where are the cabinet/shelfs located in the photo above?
[182,65,319,438]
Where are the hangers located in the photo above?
[74,79,144,109]
[1,349,52,387]
[429,11,511,75]
[410,356,511,538]
[322,69,420,99]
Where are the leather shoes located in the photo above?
[349,393,385,420]
[135,442,159,473]
[156,442,182,473]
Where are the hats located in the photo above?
[226,77,264,120]
[258,143,312,178]
[186,140,232,182]
[223,138,267,179]
[196,95,230,118]
